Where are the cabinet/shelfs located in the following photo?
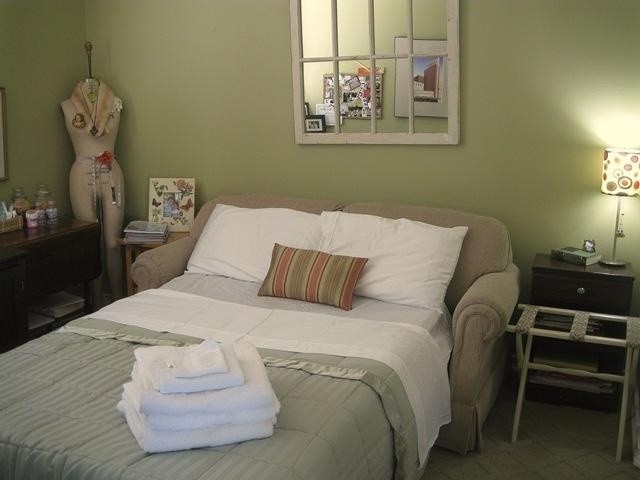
[504,303,640,462]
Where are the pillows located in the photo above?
[320,210,469,317]
[184,202,321,287]
[258,242,367,311]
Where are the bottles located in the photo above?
[7,182,61,229]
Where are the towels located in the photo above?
[115,339,281,454]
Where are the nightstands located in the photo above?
[117,227,191,297]
[530,251,637,411]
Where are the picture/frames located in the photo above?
[304,103,309,116]
[148,178,195,233]
[393,36,448,119]
[305,115,327,133]
[0,87,9,181]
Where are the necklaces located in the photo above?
[80,82,108,137]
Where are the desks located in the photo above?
[0,214,100,354]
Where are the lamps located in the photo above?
[597,147,639,268]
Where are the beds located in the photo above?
[1,192,520,478]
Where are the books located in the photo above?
[527,355,618,394]
[33,289,87,318]
[123,220,169,243]
[534,312,605,334]
[551,245,601,268]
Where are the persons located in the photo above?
[60,78,128,310]
